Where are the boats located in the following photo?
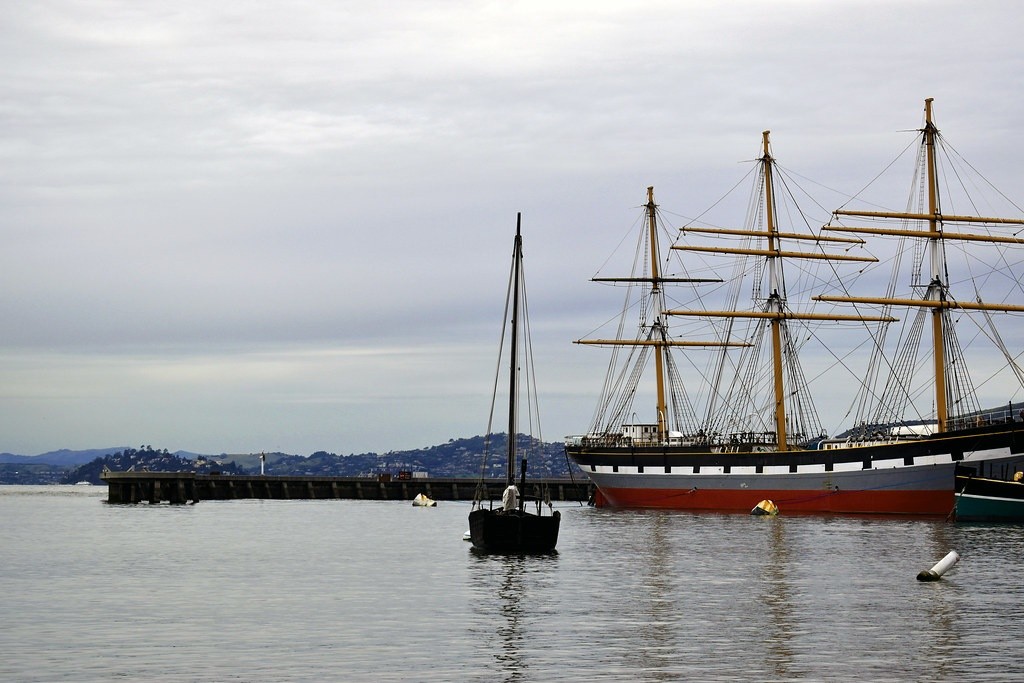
[952,460,1024,525]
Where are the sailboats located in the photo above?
[568,99,1024,525]
[470,212,560,556]
